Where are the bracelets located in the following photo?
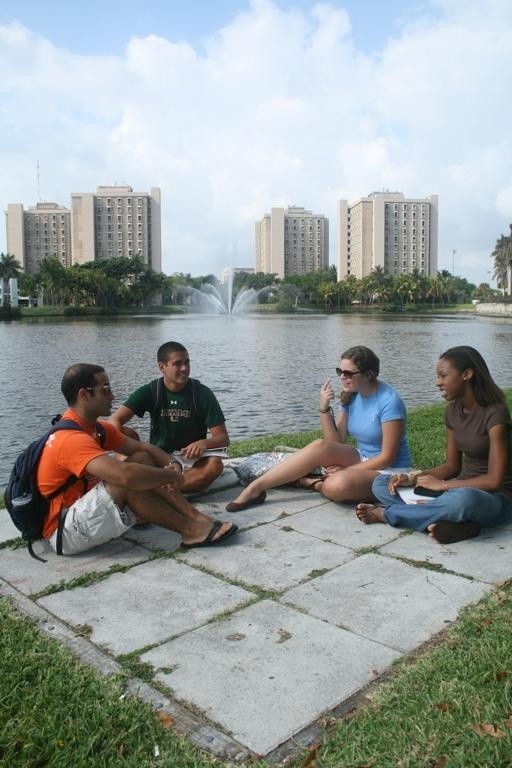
[171,460,183,474]
[318,406,335,418]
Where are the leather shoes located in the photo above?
[289,473,324,487]
[226,490,265,511]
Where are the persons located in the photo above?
[225,345,412,512]
[33,364,238,556]
[108,342,230,493]
[355,345,511,544]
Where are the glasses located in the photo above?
[336,368,360,377]
[86,385,110,394]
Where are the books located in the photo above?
[397,486,435,505]
[172,446,229,469]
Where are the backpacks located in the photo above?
[5,414,105,562]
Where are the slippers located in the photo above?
[433,521,480,543]
[181,521,237,548]
[356,503,385,523]
